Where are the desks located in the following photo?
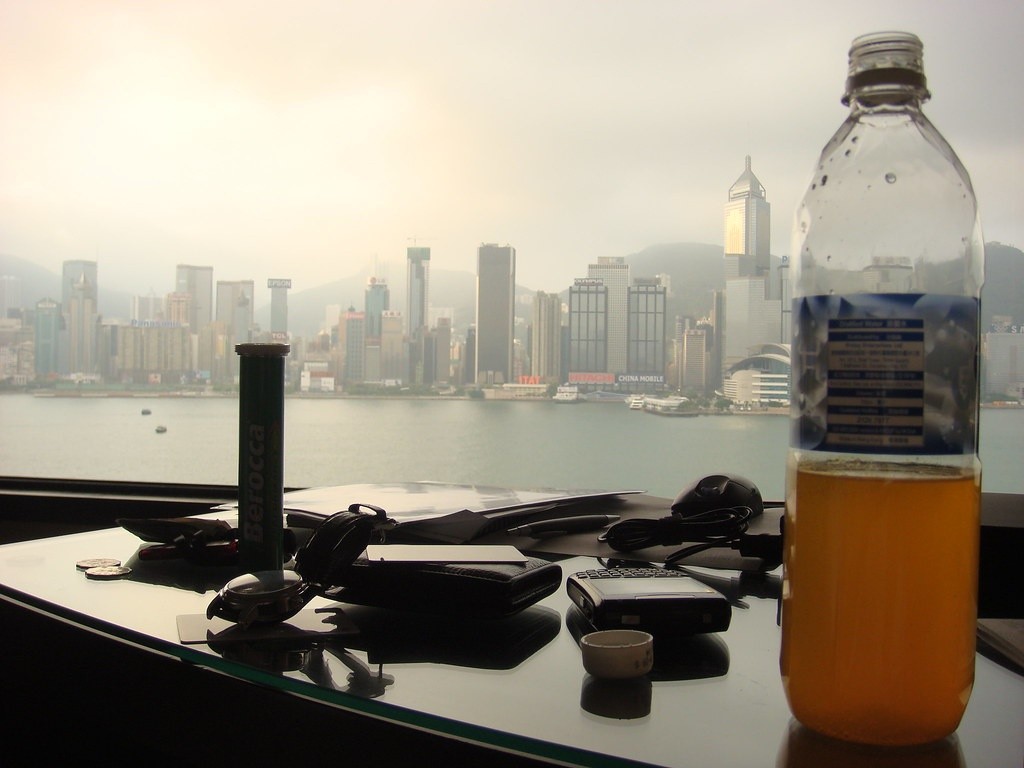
[1,486,1024,767]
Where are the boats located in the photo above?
[553,387,586,403]
[625,396,643,410]
[141,409,151,416]
[155,426,167,433]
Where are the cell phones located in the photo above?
[566,566,732,633]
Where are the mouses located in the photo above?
[669,471,764,521]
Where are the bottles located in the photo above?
[786,26,984,740]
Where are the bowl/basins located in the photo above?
[581,630,653,679]
